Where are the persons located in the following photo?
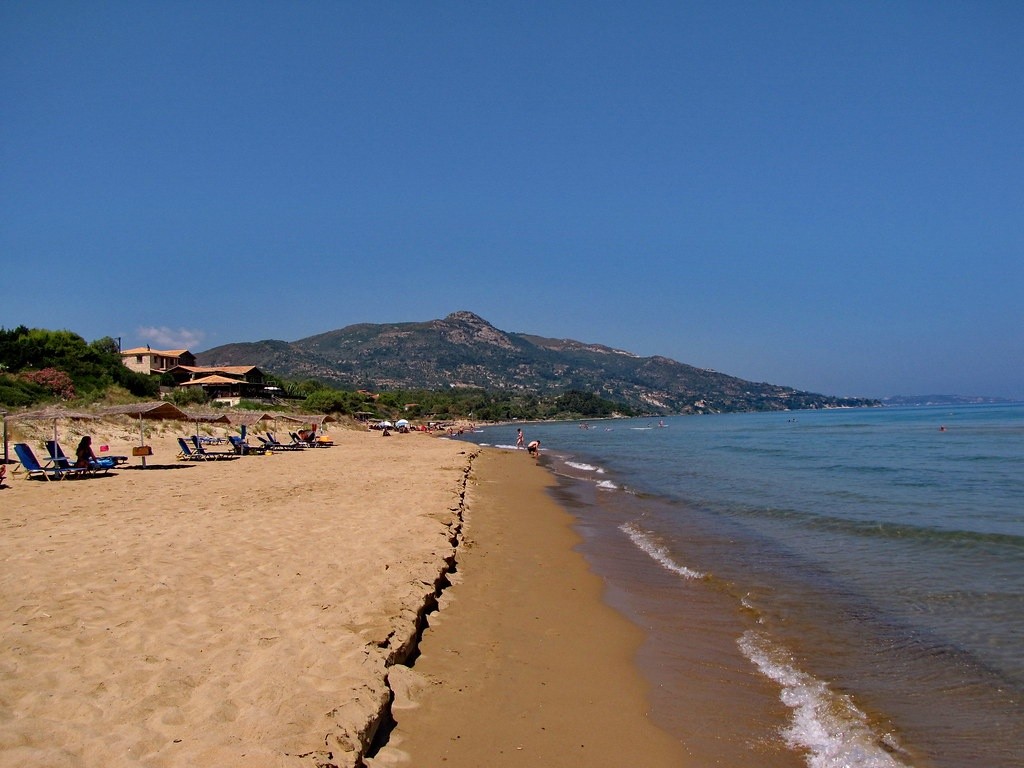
[298,429,317,442]
[516,429,540,456]
[304,429,315,442]
[658,419,663,427]
[370,422,475,436]
[579,422,589,429]
[76,436,97,474]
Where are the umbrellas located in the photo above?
[0,400,337,475]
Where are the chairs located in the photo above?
[175,432,333,462]
[10,441,127,482]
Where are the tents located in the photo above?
[379,419,408,429]
